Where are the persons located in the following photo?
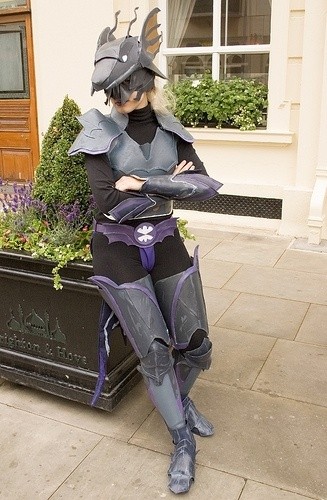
[67,8,224,494]
